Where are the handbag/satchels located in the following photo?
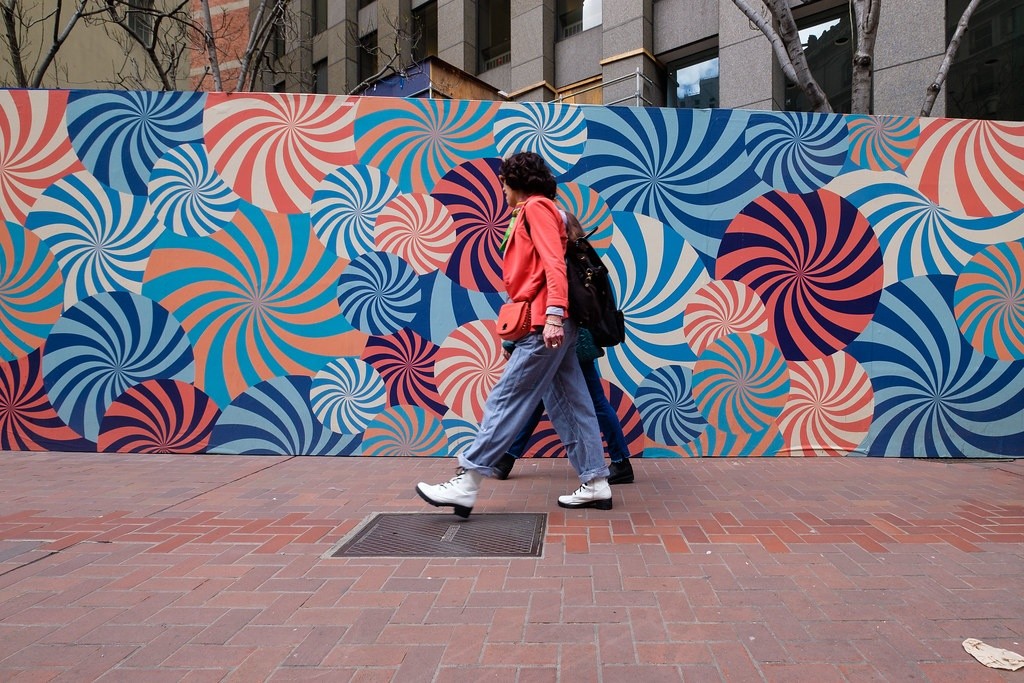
[497,299,532,341]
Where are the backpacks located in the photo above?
[563,238,625,347]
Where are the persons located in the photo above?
[491,210,634,485]
[415,151,614,518]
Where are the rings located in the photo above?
[551,344,558,348]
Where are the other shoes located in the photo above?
[492,454,516,480]
[607,459,634,484]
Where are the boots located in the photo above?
[416,467,480,519]
[558,477,613,510]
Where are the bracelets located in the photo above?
[545,320,565,327]
